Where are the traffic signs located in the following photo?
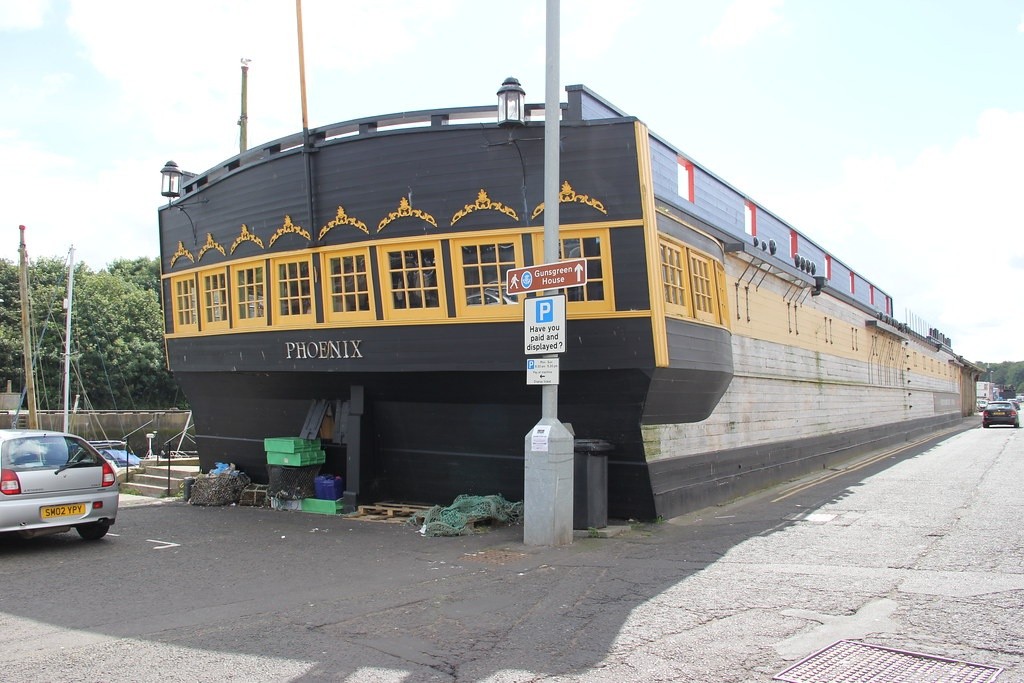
[506,258,588,295]
[526,357,560,386]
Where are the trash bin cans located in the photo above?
[574,438,616,530]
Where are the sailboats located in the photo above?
[0,223,198,479]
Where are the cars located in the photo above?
[981,401,1020,429]
[0,427,121,541]
[977,397,1021,413]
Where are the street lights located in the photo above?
[989,371,994,401]
